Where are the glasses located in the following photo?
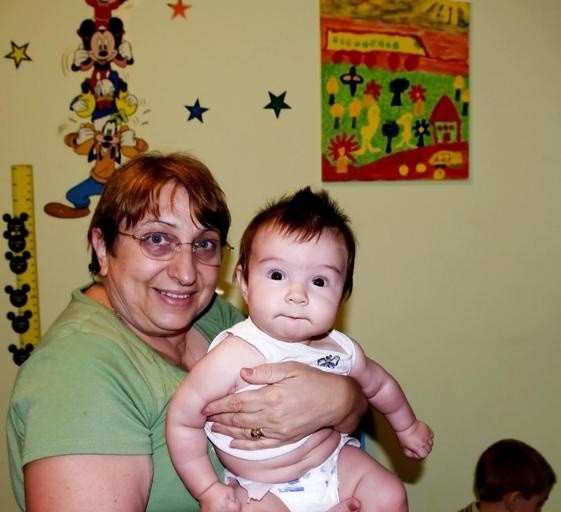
[116,229,233,268]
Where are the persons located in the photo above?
[166,185,435,511]
[4,148,372,511]
[455,437,557,512]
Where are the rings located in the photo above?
[250,428,268,440]
